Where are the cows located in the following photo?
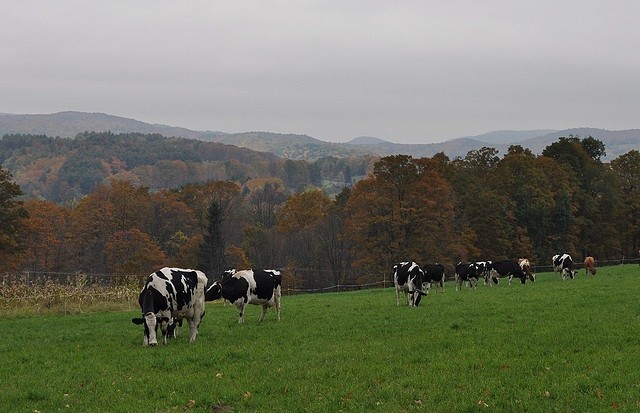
[205,269,282,324]
[419,263,445,296]
[484,261,528,287]
[455,261,488,291]
[220,269,238,310]
[552,253,579,280]
[157,317,183,345]
[517,257,535,284]
[393,261,427,308]
[583,256,596,275]
[132,267,209,346]
